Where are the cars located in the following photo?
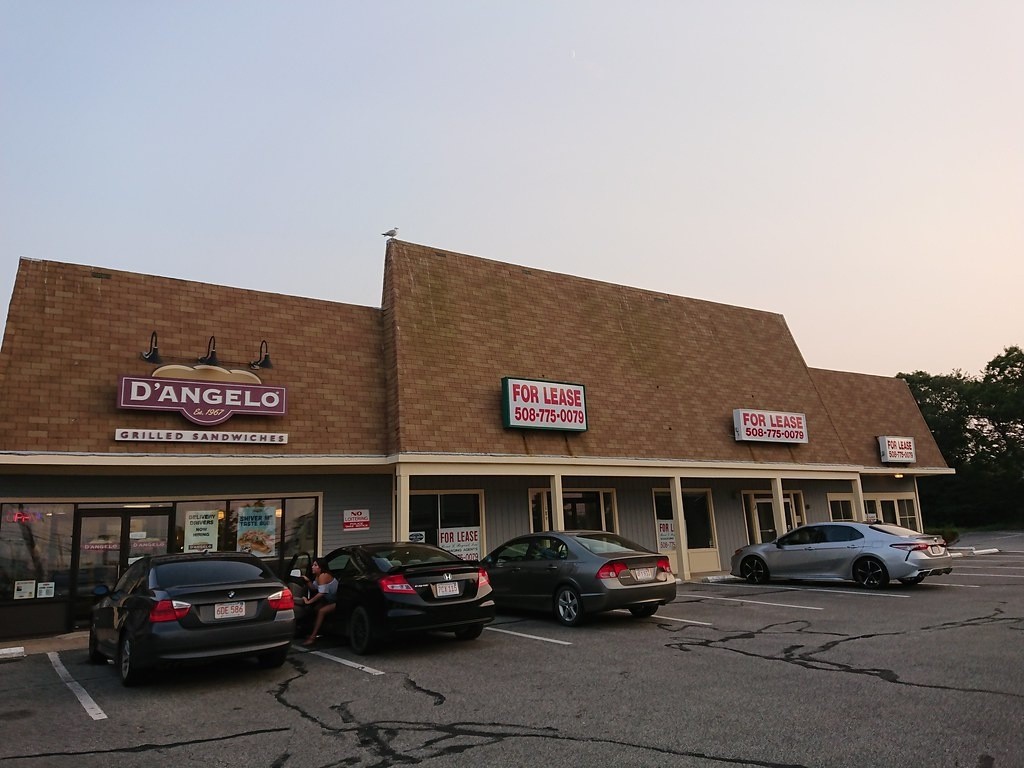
[729,520,953,591]
[88,551,298,688]
[475,530,678,626]
[279,541,496,656]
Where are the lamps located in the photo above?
[198,335,220,366]
[250,340,273,370]
[276,508,282,517]
[218,511,224,520]
[142,331,162,364]
[894,474,904,478]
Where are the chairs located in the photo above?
[390,561,402,566]
[409,559,421,564]
[428,557,443,562]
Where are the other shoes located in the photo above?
[302,639,313,645]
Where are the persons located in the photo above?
[301,558,339,644]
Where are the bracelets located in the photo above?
[306,579,310,583]
